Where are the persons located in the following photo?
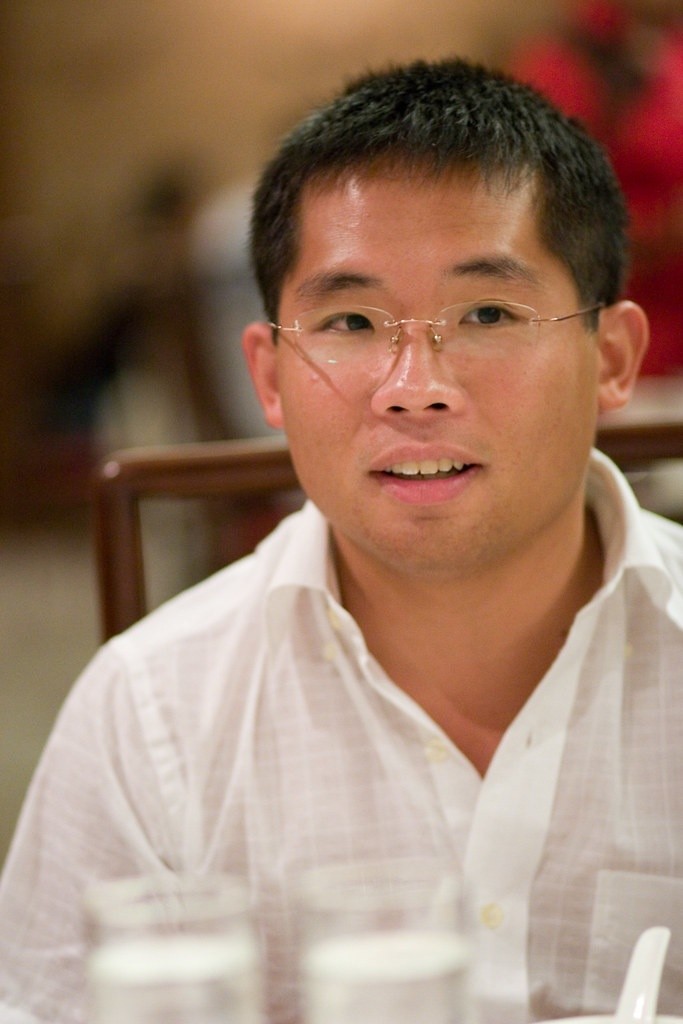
[0,62,683,1023]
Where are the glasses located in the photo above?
[270,302,603,367]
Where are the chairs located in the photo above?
[96,411,683,645]
